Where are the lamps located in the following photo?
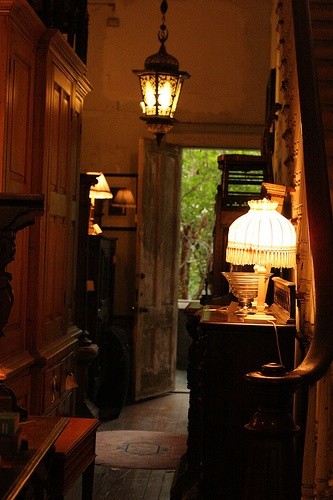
[132,0,191,147]
[85,172,113,235]
[225,198,296,320]
[112,189,136,215]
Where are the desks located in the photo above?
[197,304,295,500]
[18,417,101,500]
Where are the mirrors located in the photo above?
[99,174,139,231]
